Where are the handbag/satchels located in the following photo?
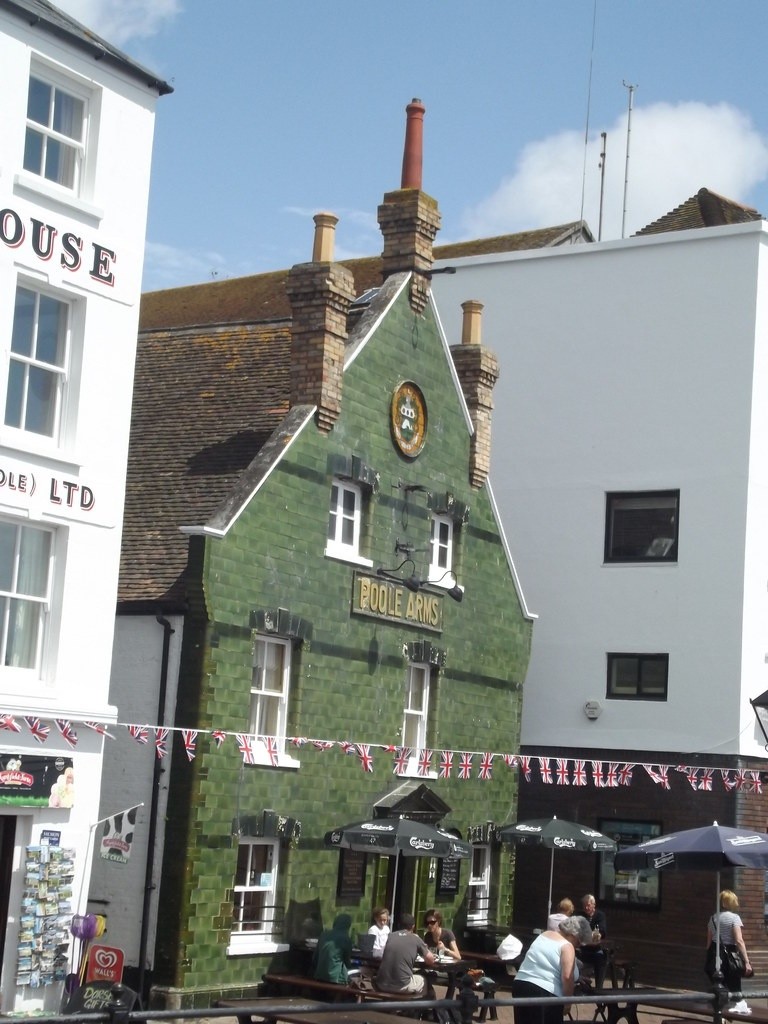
[724,952,754,979]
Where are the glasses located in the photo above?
[422,920,438,927]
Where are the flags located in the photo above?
[382,743,495,783]
[289,736,374,776]
[644,765,671,792]
[572,761,588,787]
[603,763,619,788]
[591,762,605,789]
[100,807,134,866]
[505,755,534,782]
[618,763,635,788]
[1,710,199,765]
[212,730,279,766]
[537,757,554,784]
[676,766,763,795]
[556,758,570,786]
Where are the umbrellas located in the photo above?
[492,813,617,931]
[614,818,768,972]
[324,813,472,936]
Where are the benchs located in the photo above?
[606,959,641,988]
[661,1019,713,1024]
[432,973,499,1023]
[260,974,423,1001]
[458,949,525,965]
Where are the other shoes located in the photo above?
[422,1009,446,1024]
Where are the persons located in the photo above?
[422,908,461,1004]
[372,913,434,1021]
[511,915,593,1024]
[367,905,391,963]
[703,888,752,1002]
[540,893,608,988]
[290,914,359,1004]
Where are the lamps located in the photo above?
[420,571,462,603]
[377,560,419,593]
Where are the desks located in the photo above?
[211,924,768,1024]
